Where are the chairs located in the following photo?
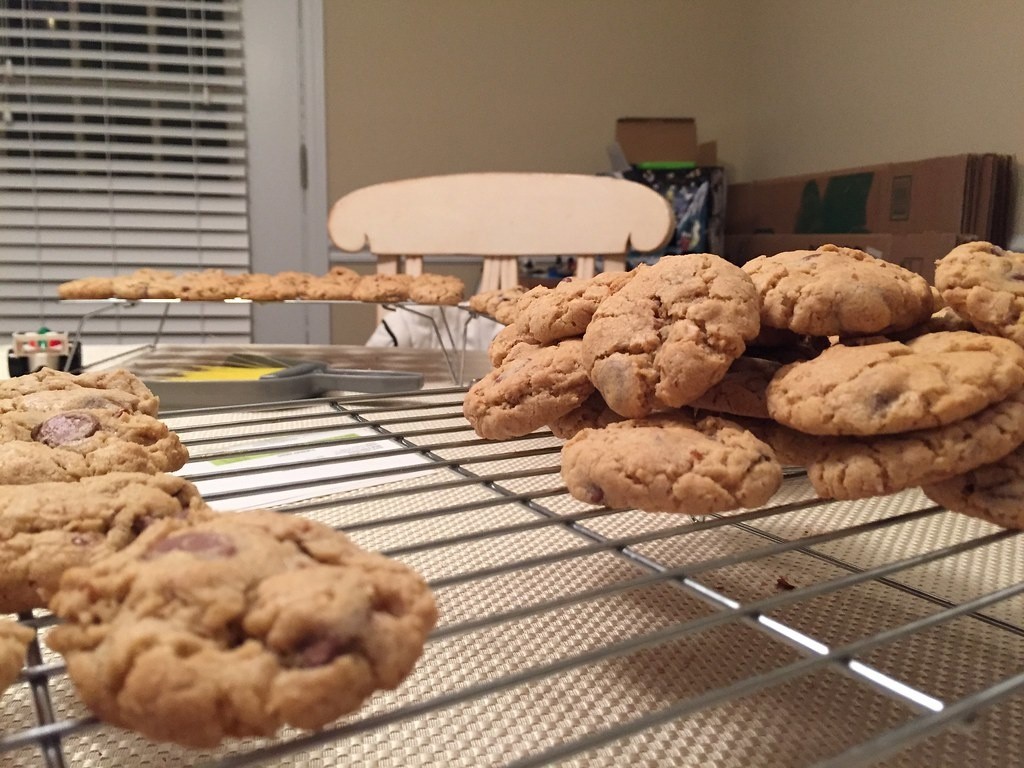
[329,172,675,350]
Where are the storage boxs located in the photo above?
[608,116,724,272]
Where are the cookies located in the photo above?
[0,243,1024,747]
